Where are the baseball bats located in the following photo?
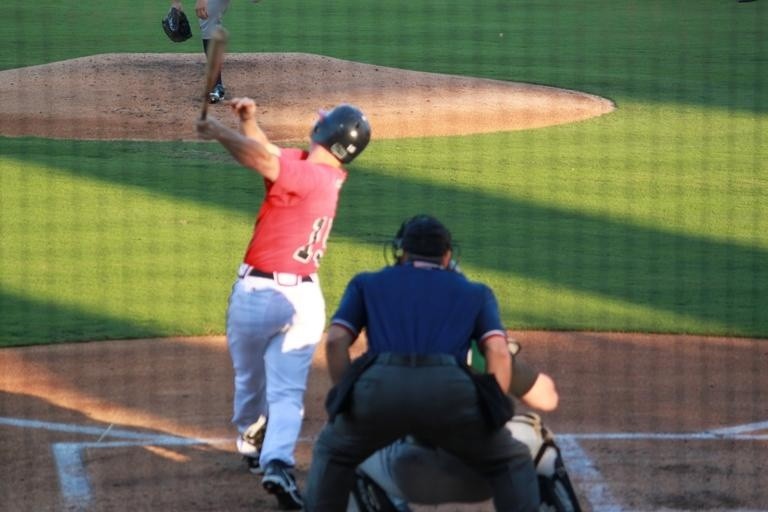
[200,25,228,120]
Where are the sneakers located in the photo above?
[207,84,225,104]
[237,448,264,476]
[261,460,304,509]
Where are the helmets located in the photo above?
[309,104,372,165]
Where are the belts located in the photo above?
[249,268,312,283]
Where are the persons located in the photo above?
[171,0,230,104]
[193,95,372,511]
[340,253,583,511]
[301,210,542,511]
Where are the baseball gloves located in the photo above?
[161,7,193,42]
